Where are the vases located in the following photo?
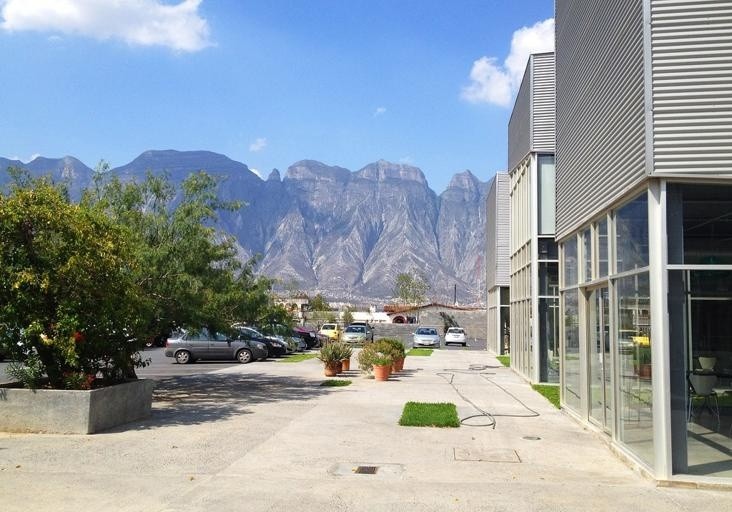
[688,357,718,396]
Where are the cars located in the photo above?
[412,326,441,349]
[166,321,340,363]
[342,322,374,347]
[445,327,467,347]
[607,330,648,353]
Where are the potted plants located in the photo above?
[316,339,406,381]
[632,346,651,380]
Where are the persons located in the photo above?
[444,323,449,337]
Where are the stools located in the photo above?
[688,389,720,423]
